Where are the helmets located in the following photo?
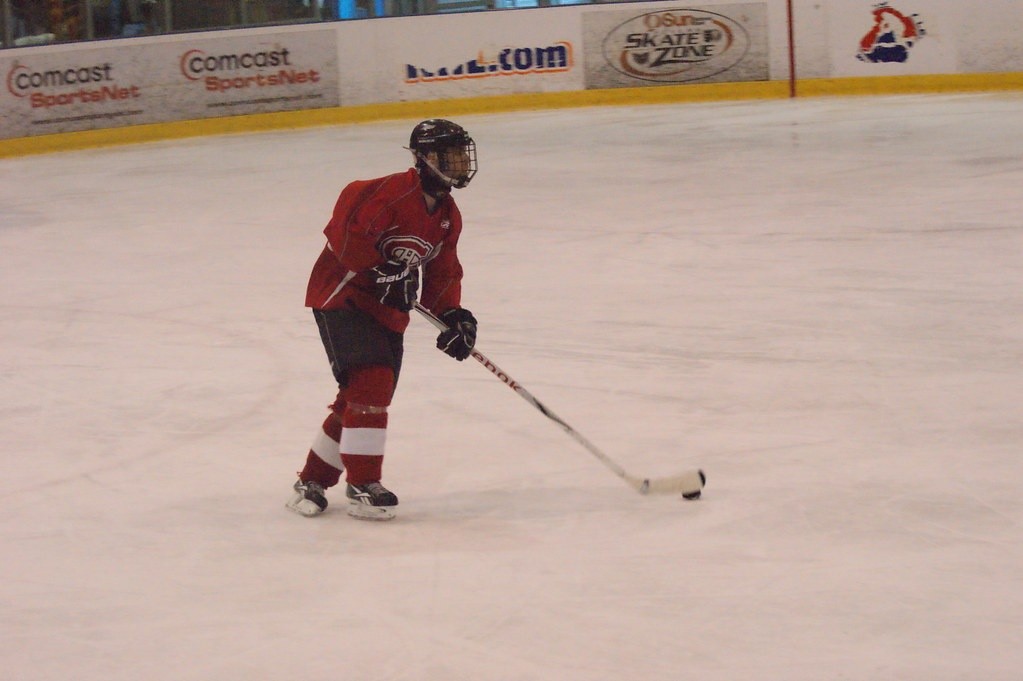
[410,119,479,188]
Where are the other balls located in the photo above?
[682,490,700,499]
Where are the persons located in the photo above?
[285,119,477,519]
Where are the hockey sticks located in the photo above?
[412,300,708,495]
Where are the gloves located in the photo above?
[369,259,419,313]
[438,307,478,362]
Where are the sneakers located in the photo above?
[346,482,398,518]
[285,478,328,515]
